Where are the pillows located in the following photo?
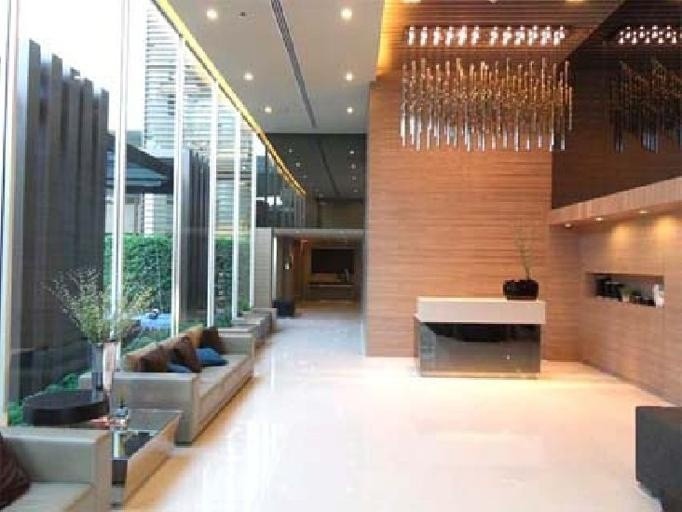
[139,326,229,373]
[1,435,30,512]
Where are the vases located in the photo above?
[503,280,539,301]
[91,342,103,392]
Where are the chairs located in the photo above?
[1,426,114,511]
[217,308,271,347]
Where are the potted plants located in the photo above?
[618,287,632,303]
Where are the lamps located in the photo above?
[399,24,575,153]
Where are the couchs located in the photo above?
[112,323,255,443]
